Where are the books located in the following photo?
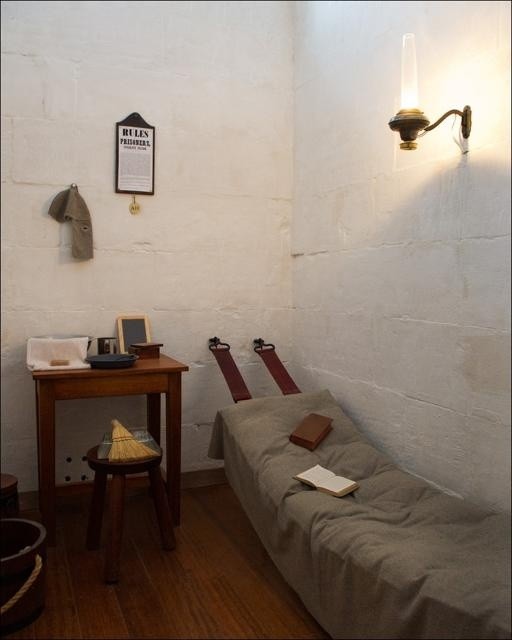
[290,413,334,451]
[293,464,360,498]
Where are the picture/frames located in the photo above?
[117,314,151,355]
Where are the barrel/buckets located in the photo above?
[0,517,49,632]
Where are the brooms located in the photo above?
[107,416,163,460]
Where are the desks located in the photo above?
[31,350,190,545]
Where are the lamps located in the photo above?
[385,32,472,154]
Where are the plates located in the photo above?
[82,353,140,369]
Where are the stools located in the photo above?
[84,442,177,583]
